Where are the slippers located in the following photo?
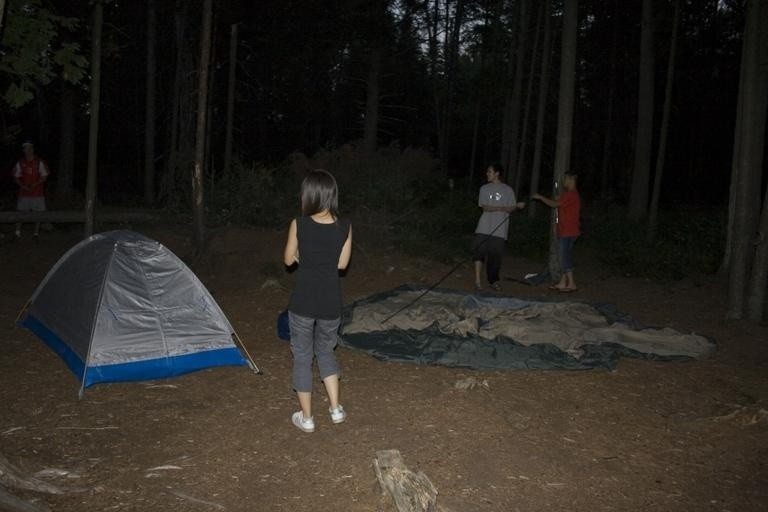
[559,288,577,293]
[549,284,560,290]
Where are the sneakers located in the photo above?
[291,410,316,432]
[31,235,41,247]
[9,232,24,242]
[489,282,503,292]
[328,404,347,424]
[475,281,483,291]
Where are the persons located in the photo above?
[529,170,582,293]
[281,169,355,434]
[470,164,525,295]
[12,142,52,239]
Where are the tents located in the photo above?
[15,228,264,397]
